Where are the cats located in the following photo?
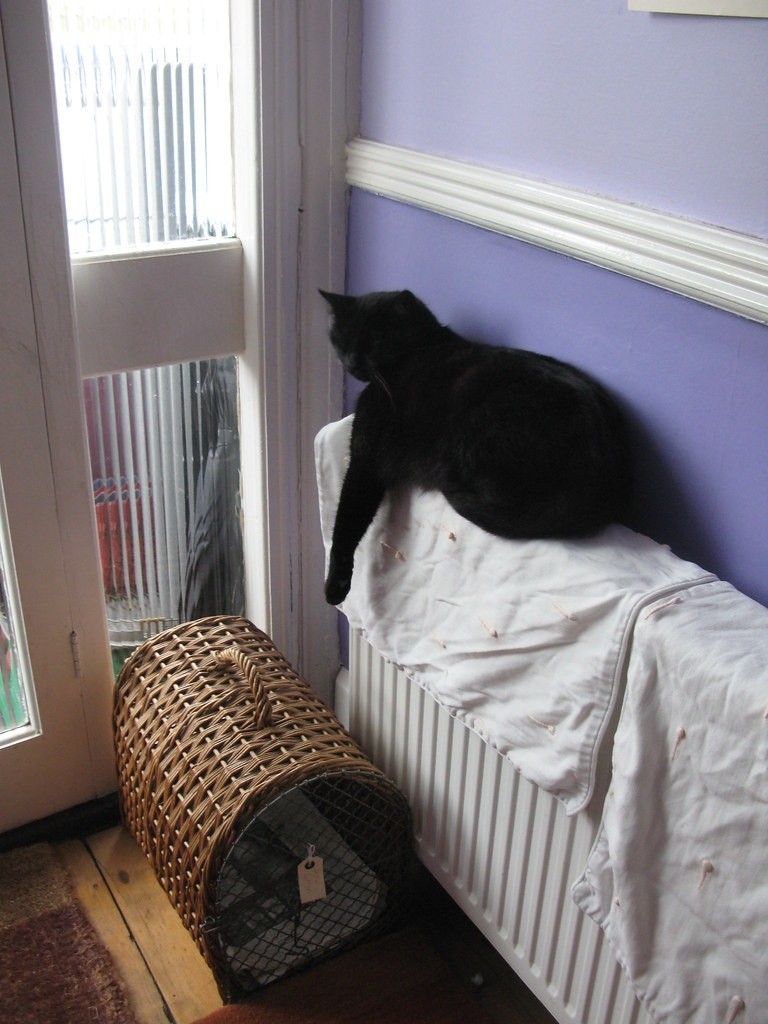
[316,284,641,606]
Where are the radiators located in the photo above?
[314,414,767,1023]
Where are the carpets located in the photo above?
[0,841,139,1024]
[191,929,493,1024]
